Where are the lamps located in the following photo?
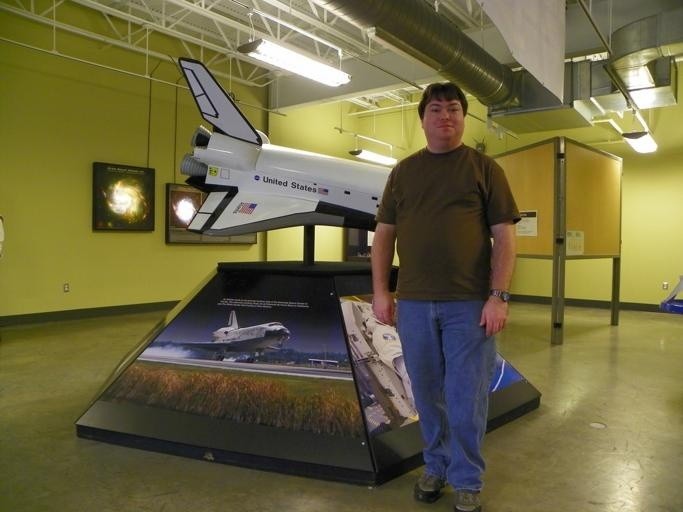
[237,7,352,89]
[622,132,658,154]
[348,134,397,166]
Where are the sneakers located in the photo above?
[414,454,452,503]
[454,489,481,512]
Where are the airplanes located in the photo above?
[179,308,294,363]
[177,56,394,236]
[308,358,339,369]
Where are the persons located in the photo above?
[370,82,522,512]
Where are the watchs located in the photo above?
[488,288,511,304]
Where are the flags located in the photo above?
[232,202,257,215]
[317,187,329,196]
